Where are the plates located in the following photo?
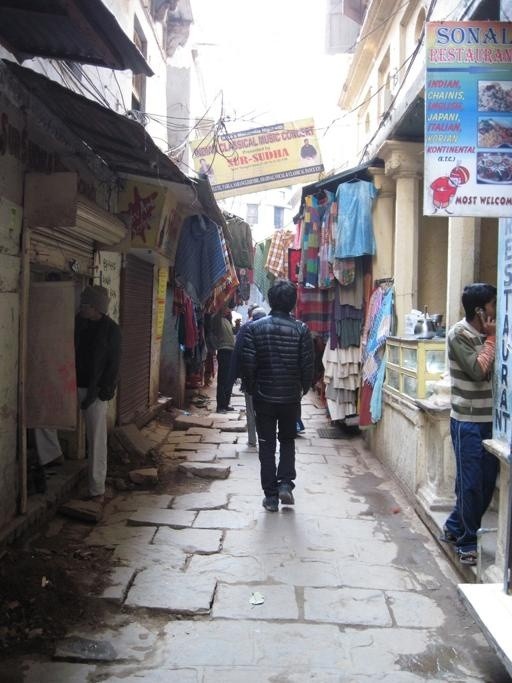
[477,156,512,184]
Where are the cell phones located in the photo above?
[475,305,487,319]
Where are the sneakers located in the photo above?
[263,497,279,512]
[216,406,234,413]
[281,491,295,509]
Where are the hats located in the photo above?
[252,307,266,321]
[80,283,109,314]
[248,302,258,312]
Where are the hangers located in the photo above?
[309,171,363,199]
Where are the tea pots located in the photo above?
[413,304,438,339]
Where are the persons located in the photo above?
[231,306,267,444]
[199,158,214,177]
[231,317,243,333]
[300,137,317,160]
[247,302,259,318]
[214,302,238,415]
[437,281,503,566]
[234,279,317,513]
[29,285,122,502]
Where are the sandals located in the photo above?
[460,545,477,565]
[440,525,458,544]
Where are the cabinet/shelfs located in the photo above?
[382,335,447,405]
